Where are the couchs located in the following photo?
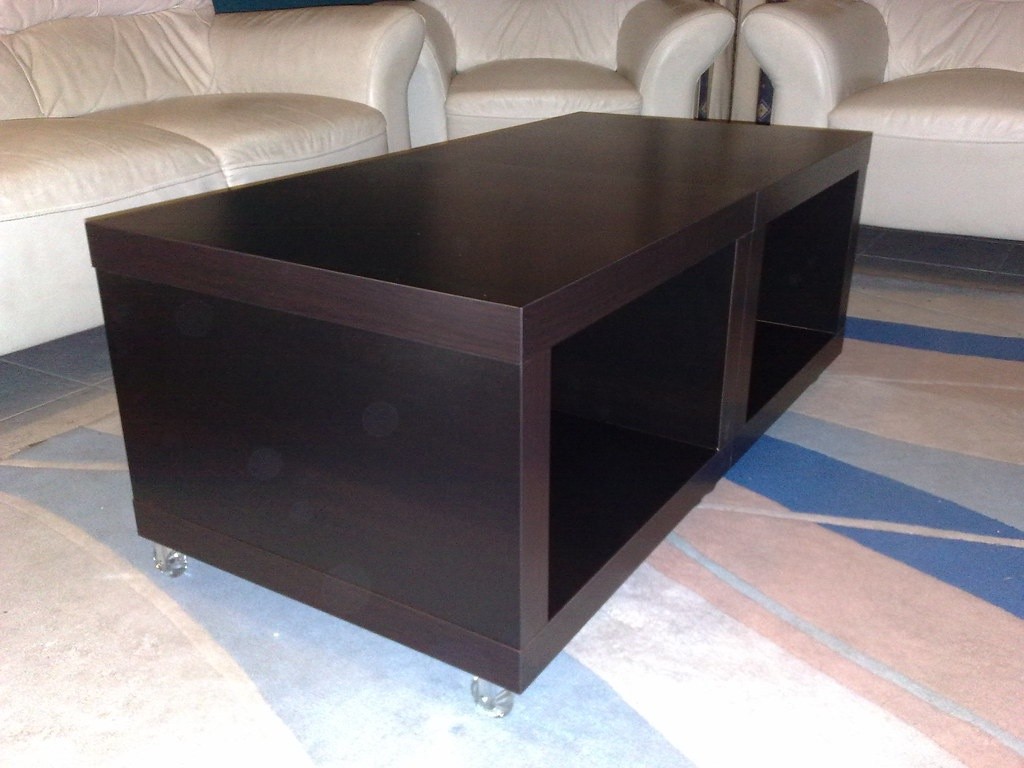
[0,0,426,357]
[372,0,736,149]
[740,0,1024,241]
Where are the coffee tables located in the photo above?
[85,110,873,694]
